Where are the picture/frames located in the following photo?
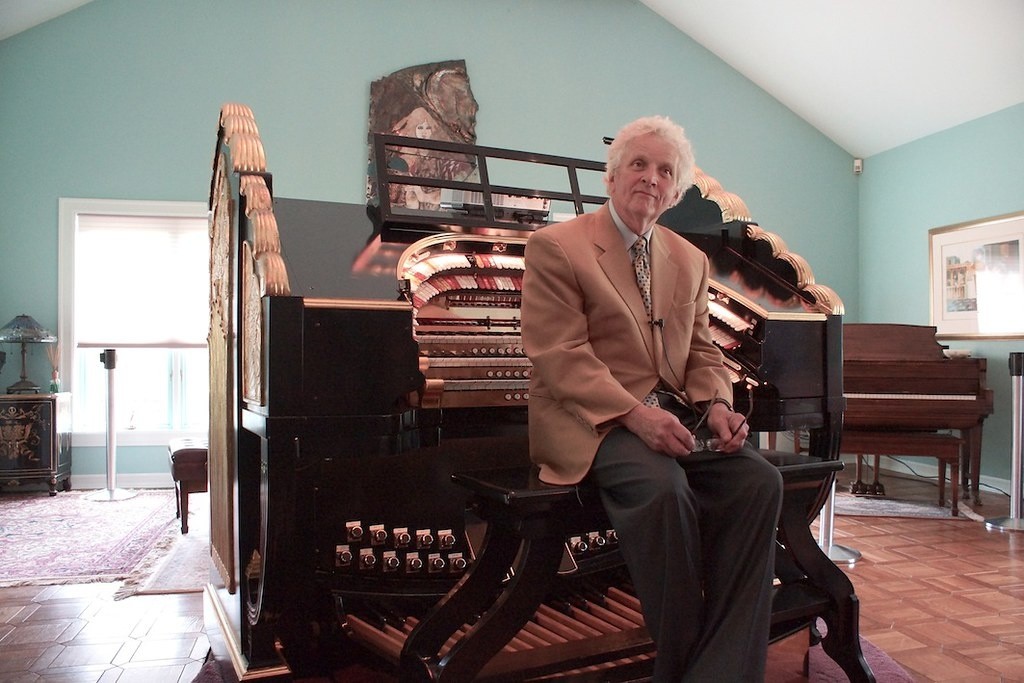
[928,210,1024,341]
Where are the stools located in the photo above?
[169,437,207,534]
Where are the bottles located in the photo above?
[49,370,60,394]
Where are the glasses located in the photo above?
[688,388,752,453]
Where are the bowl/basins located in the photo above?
[943,349,970,359]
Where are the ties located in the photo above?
[633,237,663,390]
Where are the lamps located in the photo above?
[0,314,59,394]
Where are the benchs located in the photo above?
[399,449,877,683]
[839,432,966,517]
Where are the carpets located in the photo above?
[113,493,207,600]
[0,490,184,588]
[833,493,974,521]
[195,624,915,683]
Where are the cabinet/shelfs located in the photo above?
[0,392,72,496]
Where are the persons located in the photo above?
[522,115,787,683]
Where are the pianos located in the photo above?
[202,103,849,683]
[768,323,995,515]
